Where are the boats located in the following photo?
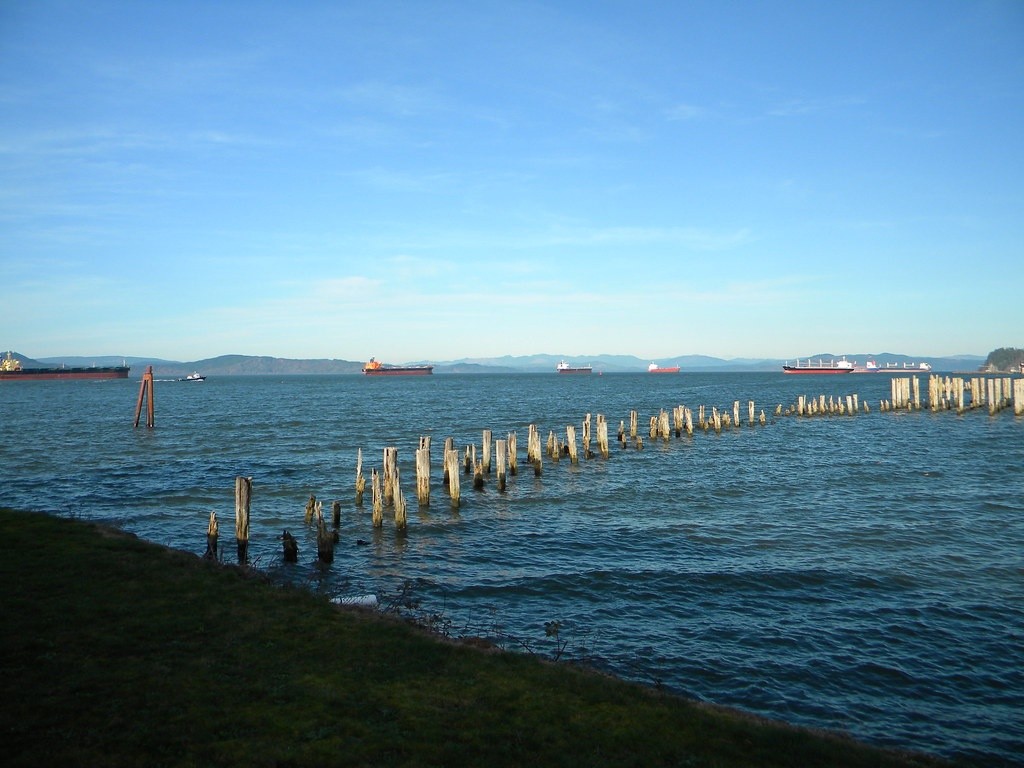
[782,359,854,373]
[556,359,593,374]
[647,362,680,373]
[177,371,207,382]
[361,357,435,375]
[0,351,131,379]
